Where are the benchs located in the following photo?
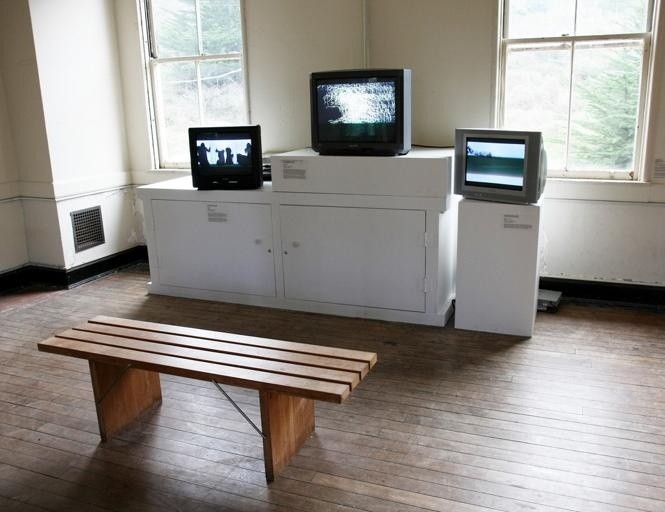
[37,313,378,486]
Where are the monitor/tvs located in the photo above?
[454,127,548,206]
[309,68,413,156]
[187,125,264,191]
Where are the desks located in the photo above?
[457,202,540,343]
[135,176,457,326]
